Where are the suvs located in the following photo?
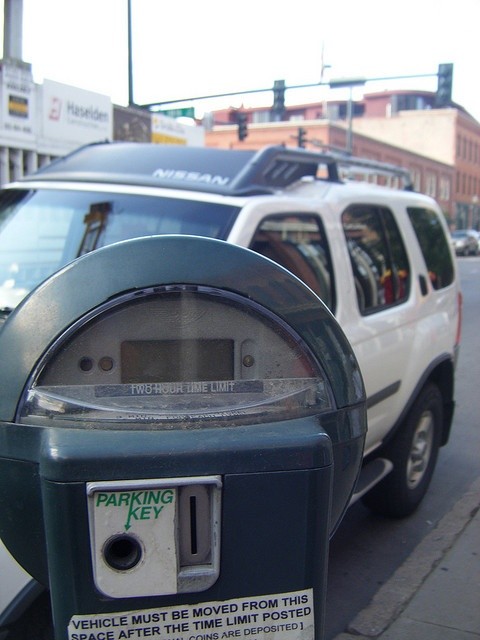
[1,140,465,518]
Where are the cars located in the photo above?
[450,229,479,255]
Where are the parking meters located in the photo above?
[0,233,368,640]
